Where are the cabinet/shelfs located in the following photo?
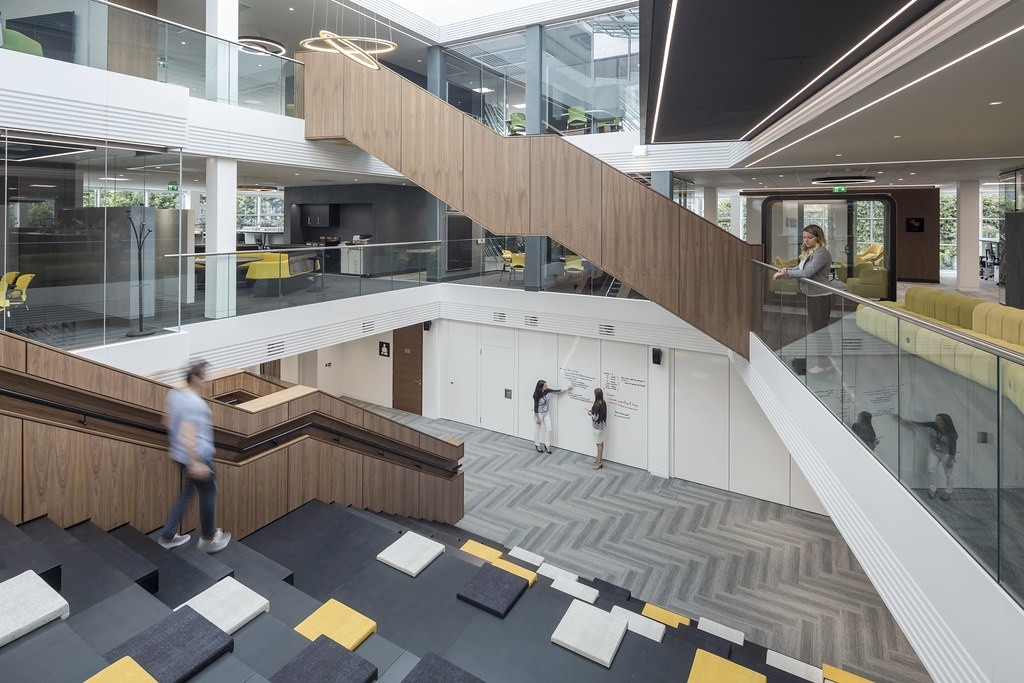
[302,204,340,227]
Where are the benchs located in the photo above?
[856,286,1024,414]
[195,252,320,298]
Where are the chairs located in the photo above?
[500,250,525,285]
[567,106,587,130]
[0,271,35,329]
[564,255,584,277]
[510,113,525,135]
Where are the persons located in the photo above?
[891,411,958,501]
[851,410,883,451]
[772,224,833,380]
[531,380,574,456]
[585,388,607,471]
[158,360,232,554]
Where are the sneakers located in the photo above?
[158,534,190,550]
[197,528,232,552]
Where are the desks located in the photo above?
[830,265,841,281]
[195,256,263,266]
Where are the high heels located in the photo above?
[545,445,553,454]
[929,491,938,499]
[593,461,603,470]
[807,364,836,380]
[592,458,598,463]
[941,493,952,500]
[535,444,544,454]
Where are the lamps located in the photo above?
[299,0,398,71]
[238,36,286,56]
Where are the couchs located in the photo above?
[768,256,798,292]
[834,244,888,298]
